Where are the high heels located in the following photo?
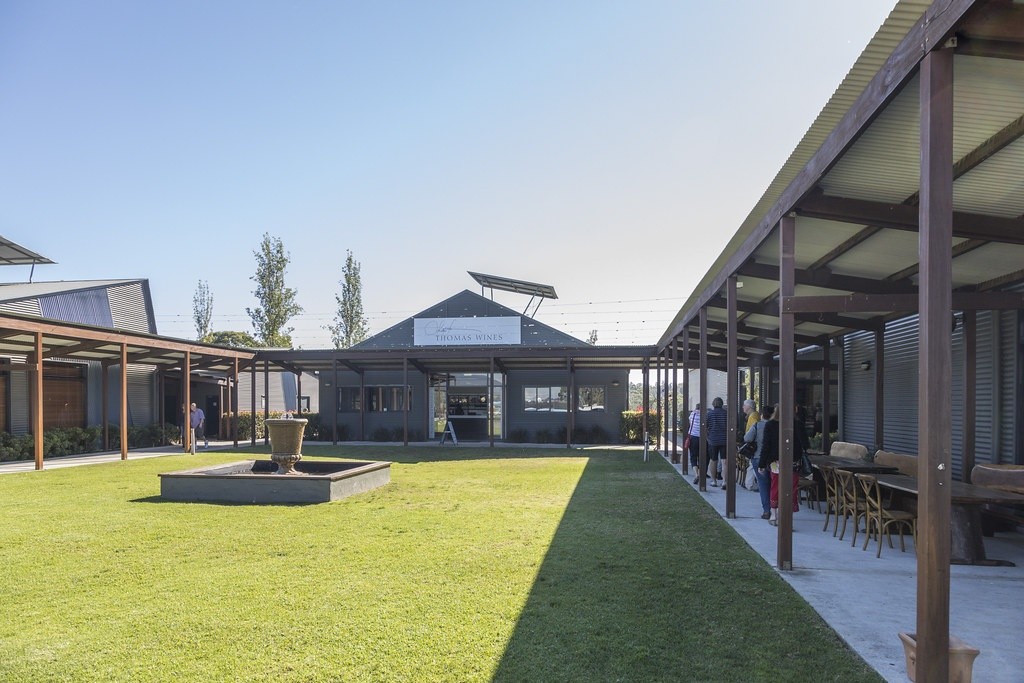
[768,518,777,526]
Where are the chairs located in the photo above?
[736,441,918,560]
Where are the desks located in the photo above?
[863,474,1024,568]
[806,448,824,455]
[807,455,898,515]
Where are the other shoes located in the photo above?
[205,440,209,449]
[710,481,718,487]
[721,483,727,490]
[761,513,771,519]
[694,476,699,484]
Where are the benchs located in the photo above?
[830,441,868,460]
[872,450,918,475]
[971,464,1024,539]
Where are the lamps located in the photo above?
[861,361,871,370]
[612,379,620,386]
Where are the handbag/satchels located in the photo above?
[799,454,813,478]
[738,442,757,459]
[685,437,691,449]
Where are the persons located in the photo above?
[177,402,208,450]
[685,396,839,529]
